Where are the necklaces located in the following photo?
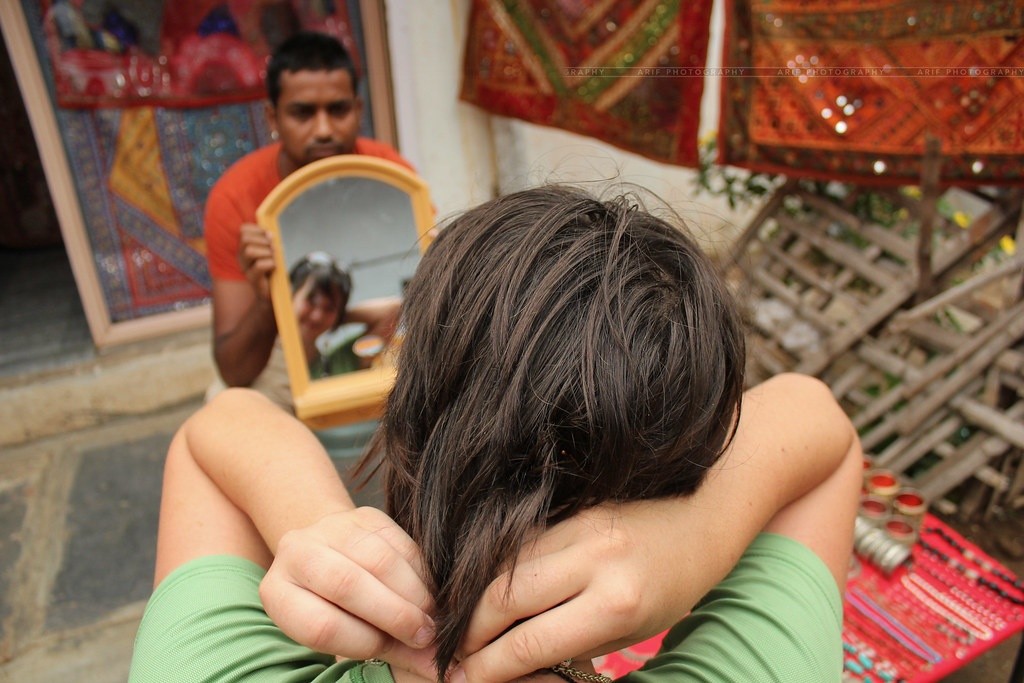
[538,664,613,683]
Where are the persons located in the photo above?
[203,30,437,416]
[130,182,866,683]
[287,251,405,381]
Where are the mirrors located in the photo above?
[280,178,424,383]
